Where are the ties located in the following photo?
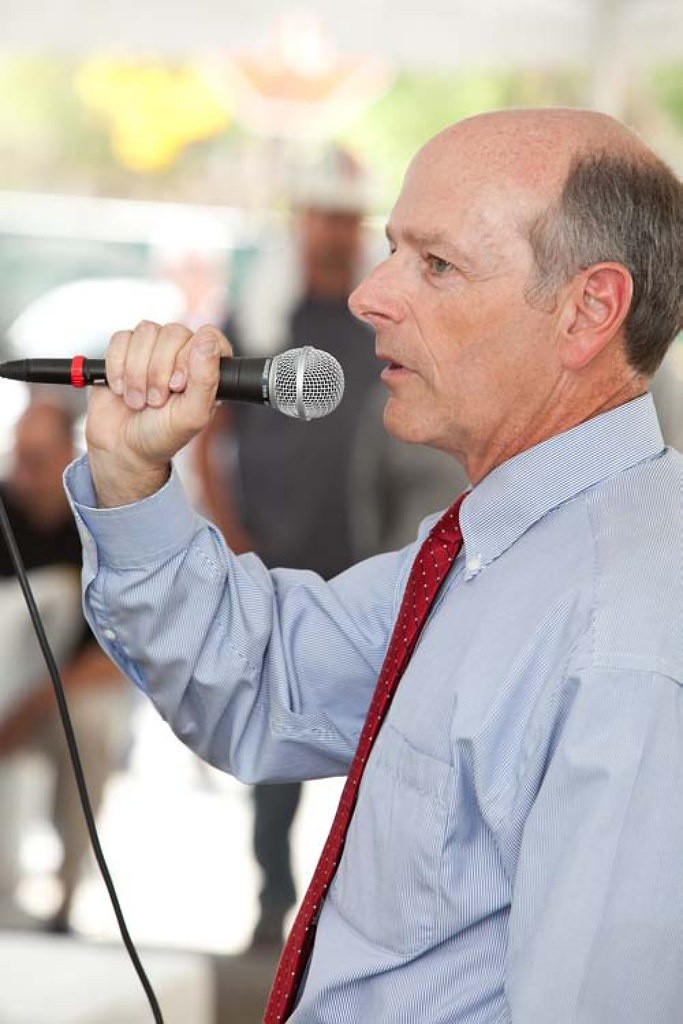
[257,484,472,1024]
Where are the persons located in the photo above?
[1,404,146,932]
[201,142,472,935]
[52,107,683,1023]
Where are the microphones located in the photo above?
[0,346,345,422]
